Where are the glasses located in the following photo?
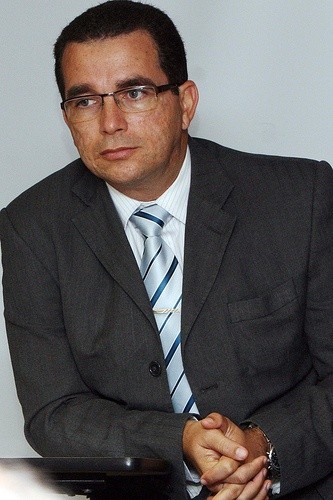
[60,83,178,124]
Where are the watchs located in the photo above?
[247,424,282,478]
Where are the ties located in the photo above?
[129,204,200,415]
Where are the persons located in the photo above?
[0,0,333,500]
